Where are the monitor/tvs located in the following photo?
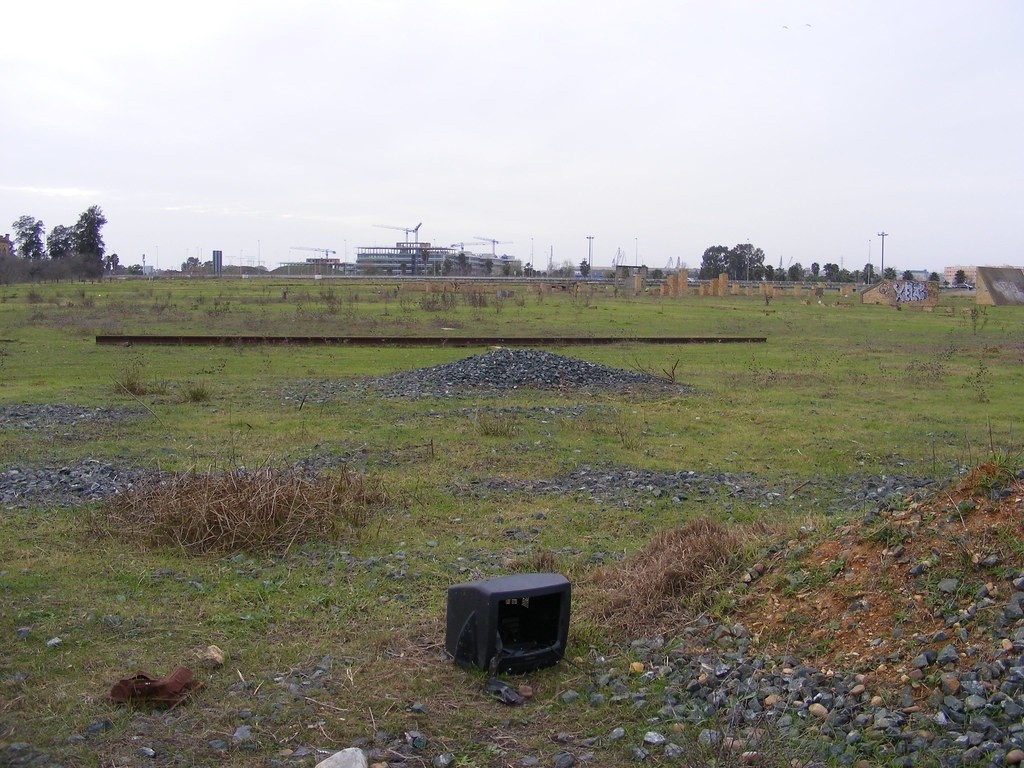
[448,573,571,675]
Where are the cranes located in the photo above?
[450,239,513,254]
[289,246,337,259]
[369,222,424,243]
[472,235,500,254]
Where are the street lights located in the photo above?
[878,233,888,282]
[746,238,750,281]
[634,237,639,267]
[585,236,595,267]
